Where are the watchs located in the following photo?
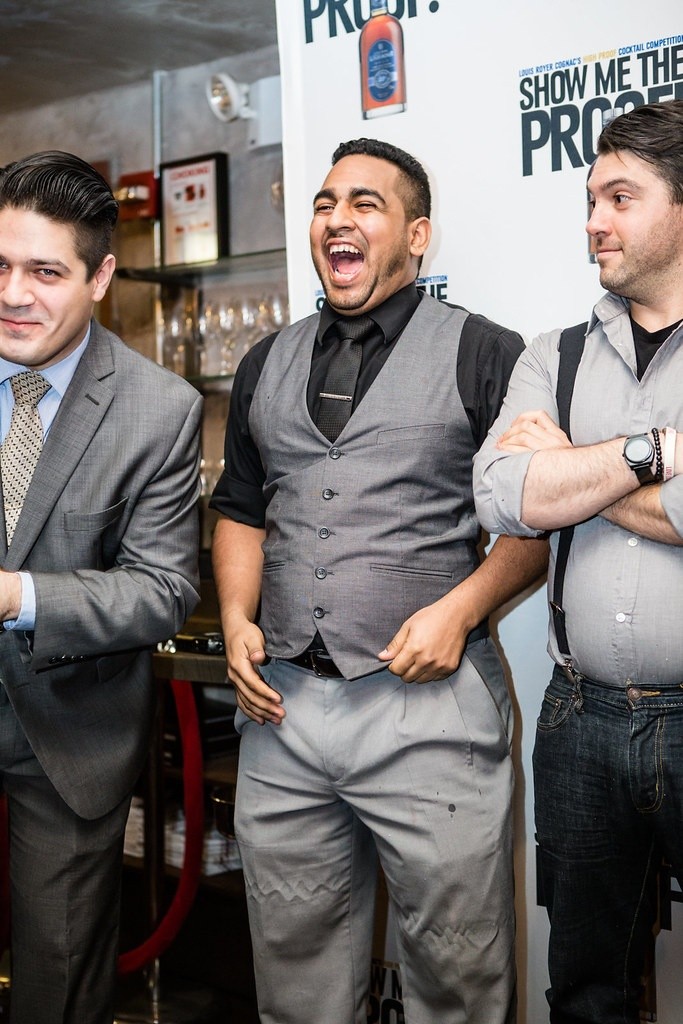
[621,431,655,487]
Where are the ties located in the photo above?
[318,317,376,444]
[0,373,52,550]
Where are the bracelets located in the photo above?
[652,426,677,483]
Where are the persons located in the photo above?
[205,139,549,1024]
[0,149,205,1024]
[474,100,683,1024]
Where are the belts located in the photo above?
[283,651,341,679]
